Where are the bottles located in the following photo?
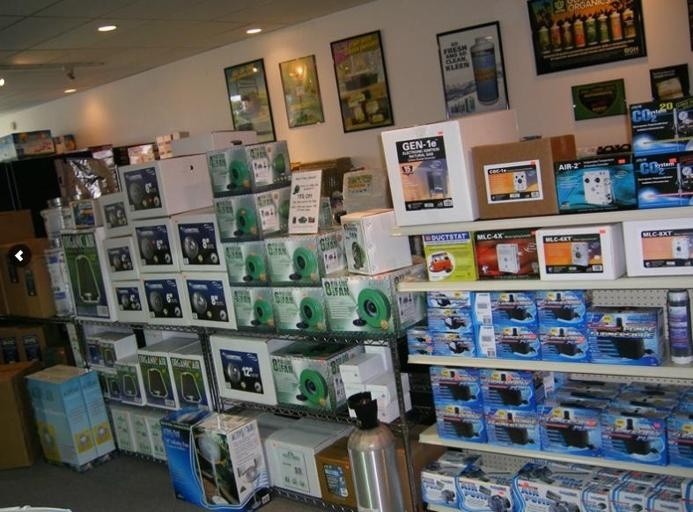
[667,290,693,364]
[539,6,637,55]
[470,35,499,106]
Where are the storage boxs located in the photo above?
[0,96,692,511]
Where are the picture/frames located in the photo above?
[278,54,325,128]
[436,21,510,120]
[223,58,276,146]
[330,30,395,133]
[528,0,647,75]
